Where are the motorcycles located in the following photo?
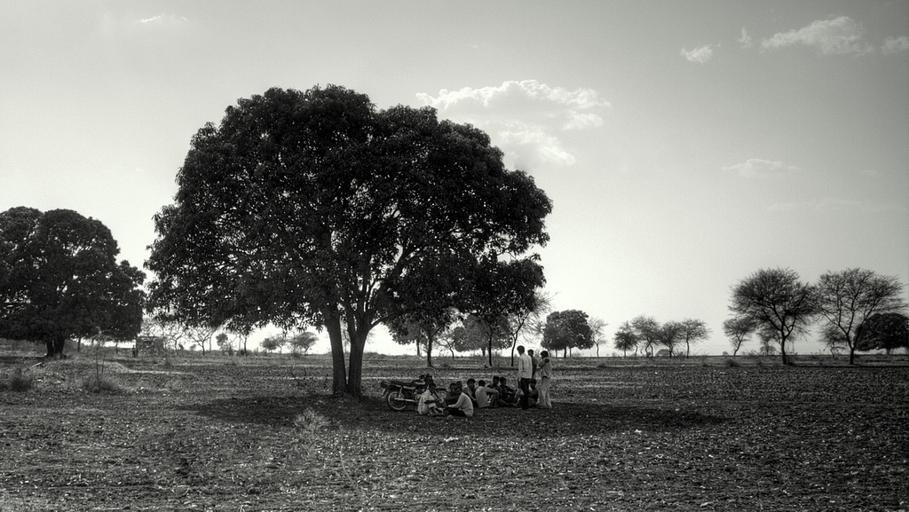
[380,373,450,412]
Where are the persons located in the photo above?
[417,346,553,418]
